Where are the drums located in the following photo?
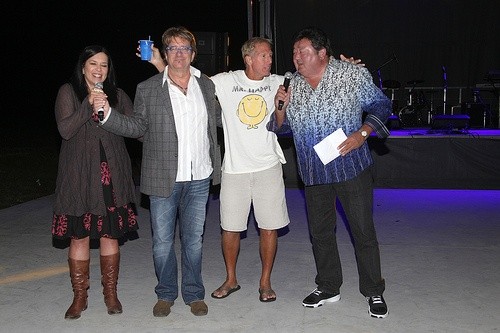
[413,96,426,106]
[399,107,418,126]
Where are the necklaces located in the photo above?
[168,75,187,95]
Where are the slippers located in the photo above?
[211,285,241,299]
[259,287,276,302]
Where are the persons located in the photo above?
[93,28,222,317]
[55,46,142,320]
[135,37,366,302]
[266,26,393,318]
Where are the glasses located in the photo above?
[165,46,192,52]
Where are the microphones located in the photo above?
[278,72,293,110]
[95,82,105,122]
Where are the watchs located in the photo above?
[358,130,368,139]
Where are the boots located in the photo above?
[100,251,123,315]
[65,255,90,319]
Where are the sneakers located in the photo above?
[153,300,174,317]
[365,296,389,318]
[189,300,208,316]
[301,286,341,308]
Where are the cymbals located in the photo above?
[382,80,400,89]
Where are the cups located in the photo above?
[140,40,154,61]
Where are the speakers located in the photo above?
[190,32,229,76]
[461,103,491,128]
[432,114,470,131]
[384,116,399,131]
